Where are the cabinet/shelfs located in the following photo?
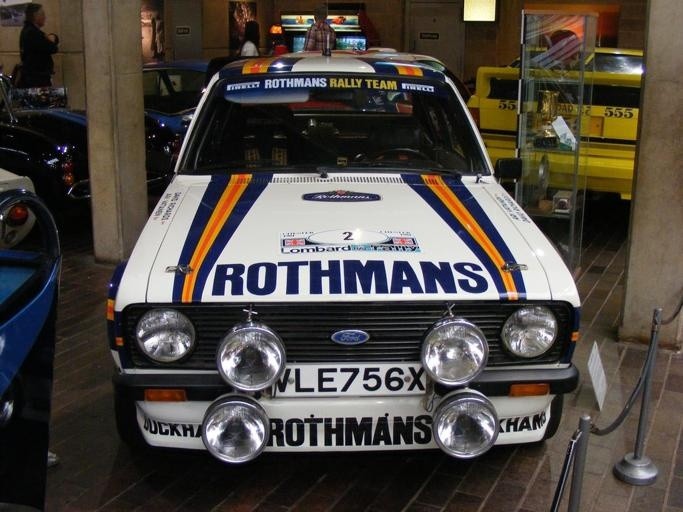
[514,10,602,283]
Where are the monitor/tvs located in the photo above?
[287,34,305,53]
[335,37,367,52]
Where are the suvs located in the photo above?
[104,46,582,465]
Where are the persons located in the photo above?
[304,5,337,51]
[240,21,261,56]
[20,3,59,89]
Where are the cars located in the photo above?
[0,63,209,251]
[467,48,645,201]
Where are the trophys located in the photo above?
[533,89,560,148]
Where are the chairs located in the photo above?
[353,117,445,169]
[220,104,301,167]
